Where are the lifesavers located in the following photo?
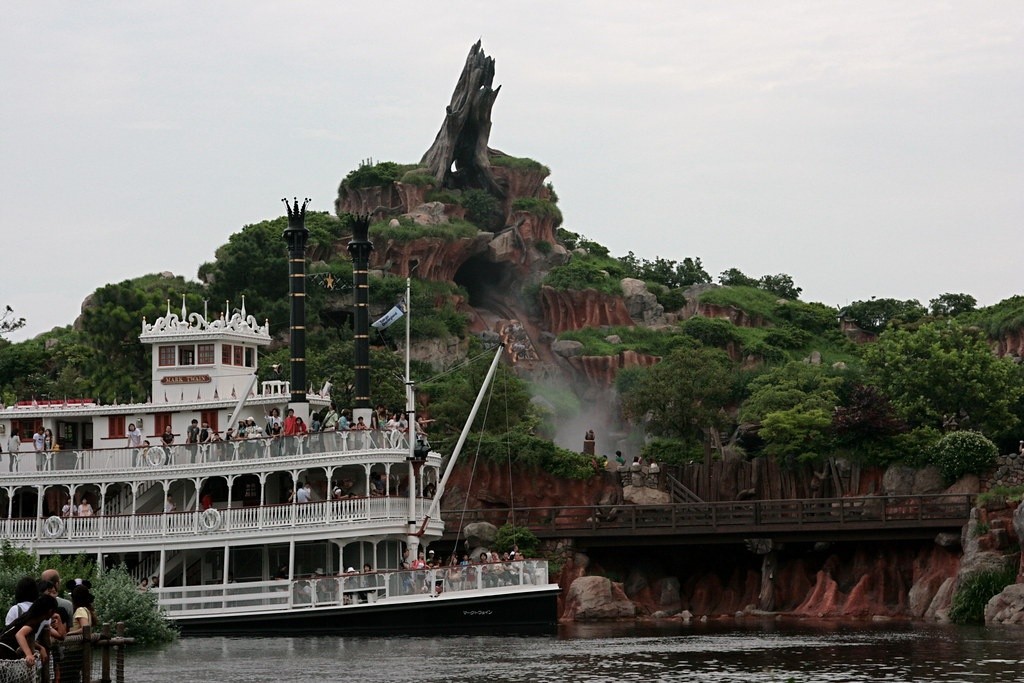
[45,515,64,540]
[146,445,167,469]
[199,509,220,532]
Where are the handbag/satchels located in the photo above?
[0,626,28,654]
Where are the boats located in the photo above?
[0,195,563,639]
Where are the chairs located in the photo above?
[320,584,336,607]
[443,567,479,592]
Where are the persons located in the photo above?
[62,496,77,517]
[601,450,661,469]
[166,493,177,511]
[7,428,21,475]
[199,491,213,510]
[78,497,94,517]
[33,427,57,471]
[285,481,437,503]
[227,572,237,583]
[275,561,376,605]
[399,547,549,596]
[0,569,99,683]
[137,576,159,590]
[123,408,437,470]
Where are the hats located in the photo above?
[315,568,324,574]
[245,417,254,421]
[347,567,355,573]
[510,551,515,555]
[341,409,352,415]
[429,550,434,554]
